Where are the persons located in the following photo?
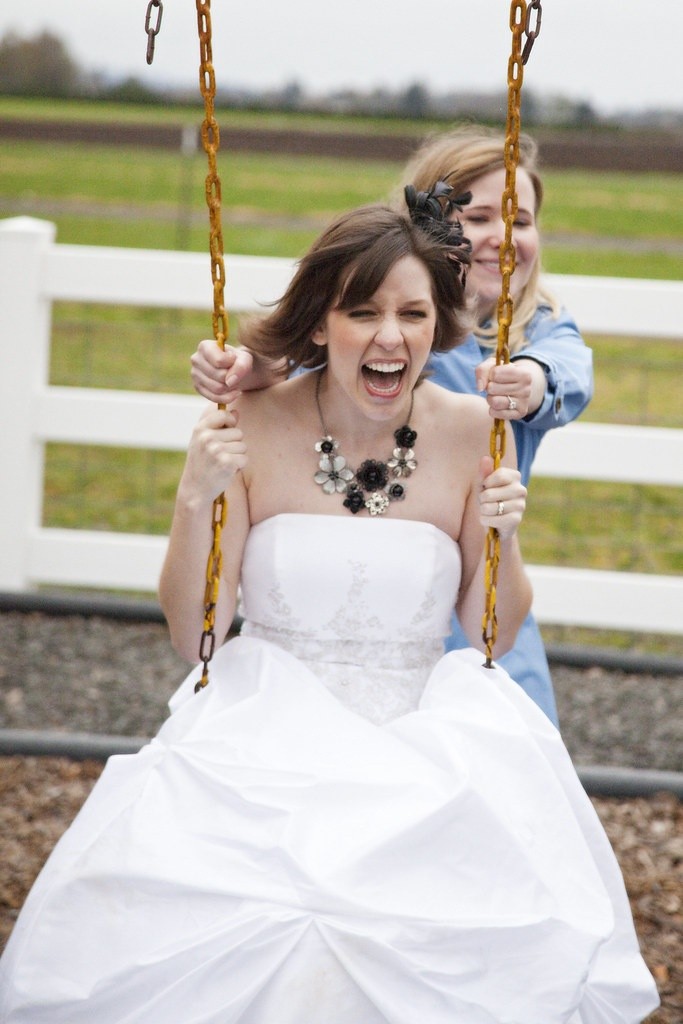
[187,127,593,732]
[0,181,659,1024]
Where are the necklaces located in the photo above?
[314,363,419,517]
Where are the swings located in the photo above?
[191,0,532,699]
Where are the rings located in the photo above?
[505,396,518,412]
[496,500,505,516]
